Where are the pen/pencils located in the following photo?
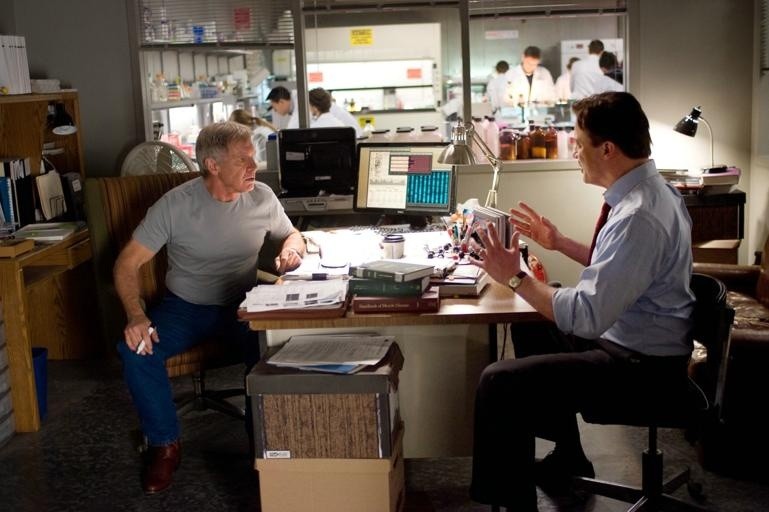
[136,323,157,355]
[281,273,349,280]
[447,224,473,251]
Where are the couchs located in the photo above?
[690,235,768,486]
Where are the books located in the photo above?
[704,183,738,195]
[473,204,512,251]
[0,155,33,239]
[343,252,488,314]
[266,333,395,377]
[702,165,743,185]
[11,222,86,241]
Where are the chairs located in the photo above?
[572,274,734,511]
[98,175,279,452]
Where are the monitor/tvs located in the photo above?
[278,127,357,197]
[353,142,458,230]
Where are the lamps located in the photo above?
[673,104,714,170]
[438,116,501,212]
[46,103,79,135]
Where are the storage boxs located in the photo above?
[254,421,405,511]
[246,341,404,459]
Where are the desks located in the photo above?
[239,224,552,512]
[283,195,450,232]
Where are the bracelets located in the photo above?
[290,247,304,259]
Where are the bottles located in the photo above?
[363,119,376,137]
[555,126,577,160]
[150,70,170,102]
[497,119,557,162]
[458,115,500,163]
[342,96,357,114]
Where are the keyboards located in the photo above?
[350,224,430,238]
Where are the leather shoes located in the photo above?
[142,440,182,495]
[534,452,594,487]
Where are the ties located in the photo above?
[588,202,612,269]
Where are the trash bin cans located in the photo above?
[31,346,49,421]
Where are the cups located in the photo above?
[382,233,407,260]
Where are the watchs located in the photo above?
[506,270,528,292]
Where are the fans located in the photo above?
[121,139,195,176]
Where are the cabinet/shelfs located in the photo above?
[0,88,95,432]
[674,184,746,263]
[145,39,256,147]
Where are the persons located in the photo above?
[113,117,308,499]
[230,87,359,161]
[488,40,623,124]
[467,88,696,512]
